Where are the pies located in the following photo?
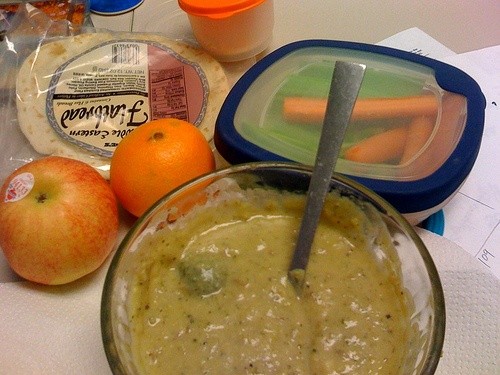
[16,32,232,178]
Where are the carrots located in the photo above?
[283,82,469,210]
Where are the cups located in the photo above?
[178,0,276,63]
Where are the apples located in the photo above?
[0,156,119,285]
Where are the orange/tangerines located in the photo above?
[110,118,216,220]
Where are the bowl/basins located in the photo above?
[101,161,445,375]
